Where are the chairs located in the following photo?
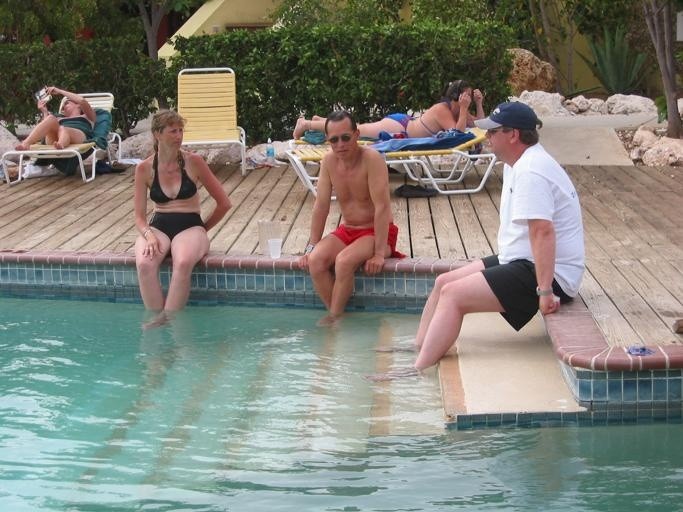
[176,67,248,176]
[284,124,495,202]
[0,93,122,187]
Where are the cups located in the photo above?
[256,218,283,260]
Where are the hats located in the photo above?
[474,101,536,129]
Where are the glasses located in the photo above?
[488,127,513,133]
[325,129,355,143]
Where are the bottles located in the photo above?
[266,137,275,164]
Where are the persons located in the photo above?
[364,102,585,382]
[293,79,486,140]
[298,111,406,328]
[15,84,96,151]
[131,108,233,330]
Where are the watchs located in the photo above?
[306,244,315,252]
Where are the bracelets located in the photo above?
[143,229,152,239]
[535,286,553,297]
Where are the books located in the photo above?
[35,85,53,105]
